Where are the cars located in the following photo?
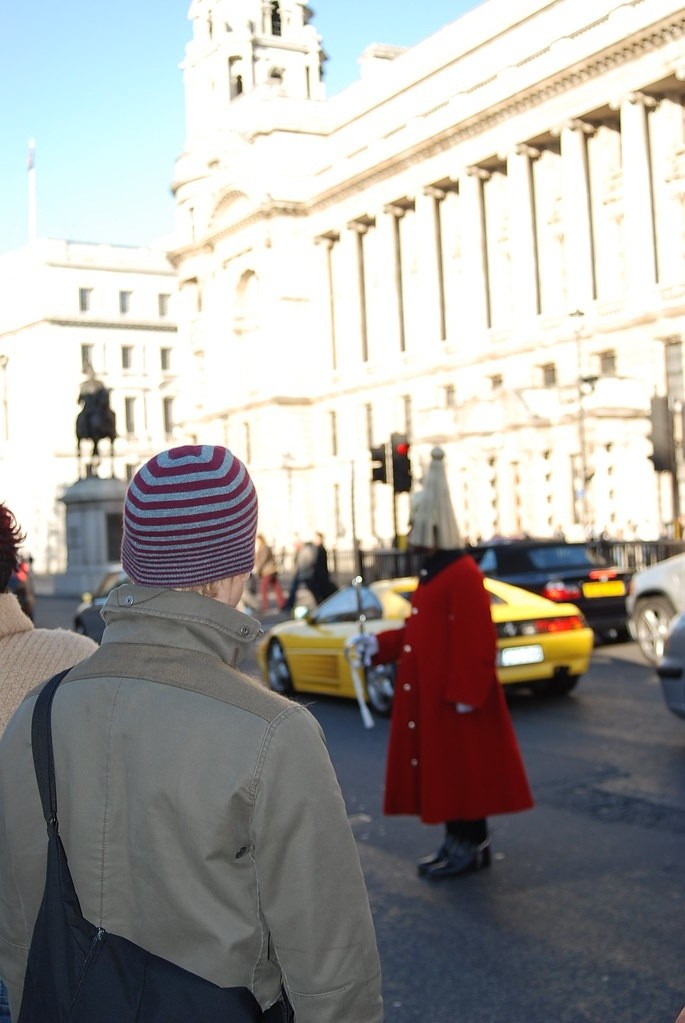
[70,568,130,644]
[624,551,685,727]
[252,574,593,719]
[9,555,38,622]
[414,539,634,646]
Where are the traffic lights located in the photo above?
[370,442,390,485]
[389,432,413,492]
[642,396,671,472]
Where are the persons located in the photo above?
[0,444,383,1023]
[78,365,116,438]
[355,449,534,880]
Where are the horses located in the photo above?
[76,388,116,457]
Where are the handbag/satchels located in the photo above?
[14,830,264,1023]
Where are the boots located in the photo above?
[418,819,489,880]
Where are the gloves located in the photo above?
[353,635,378,667]
[457,702,473,713]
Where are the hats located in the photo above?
[120,445,257,588]
[407,448,461,552]
